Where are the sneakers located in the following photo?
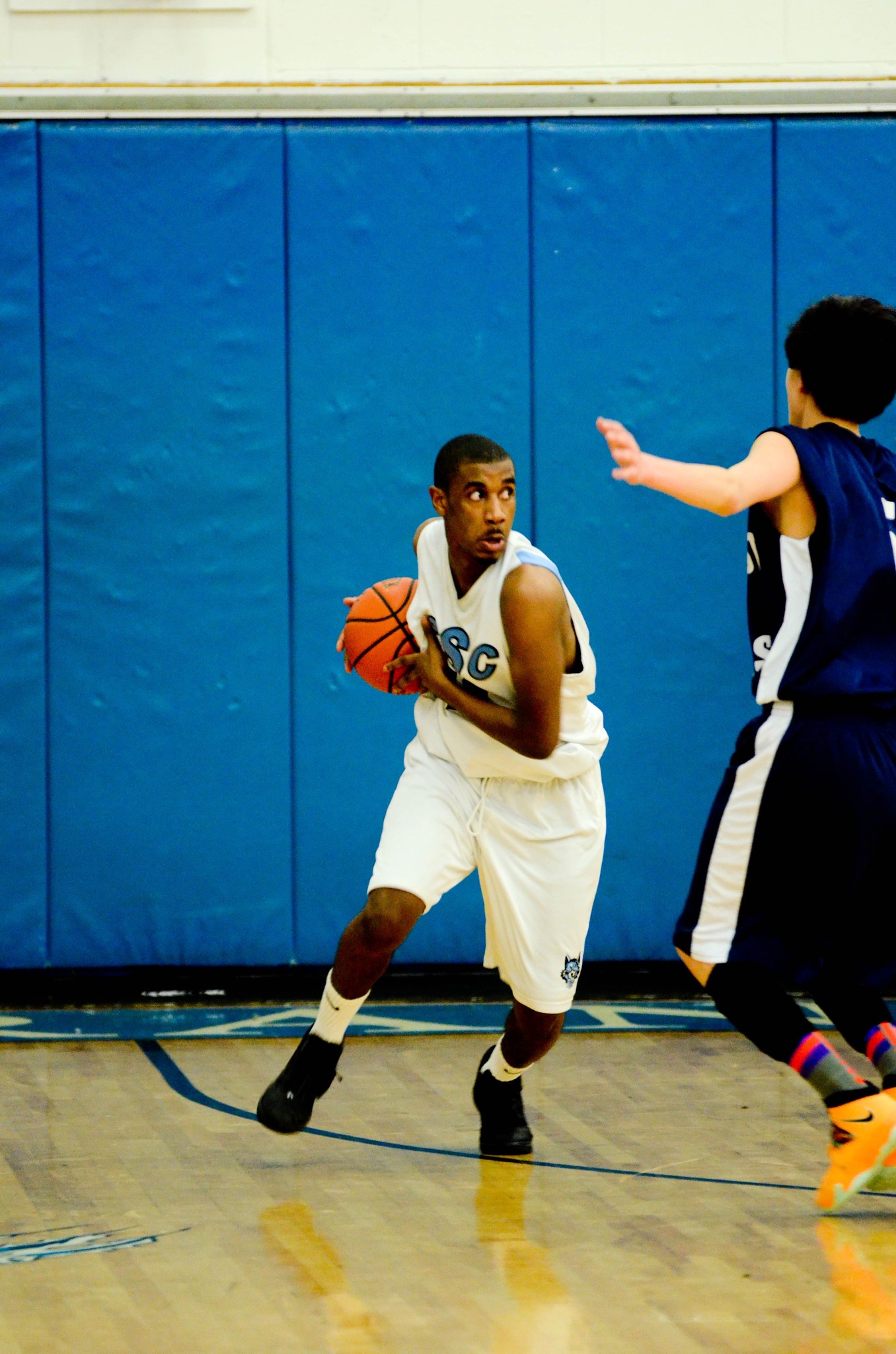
[261,1204,342,1296]
[256,1024,345,1135]
[817,1217,896,1336]
[473,1045,533,1156]
[474,1159,531,1244]
[868,1074,896,1194]
[814,1079,896,1212]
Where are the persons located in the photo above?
[596,296,896,1211]
[256,434,609,1154]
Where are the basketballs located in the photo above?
[344,577,428,695]
